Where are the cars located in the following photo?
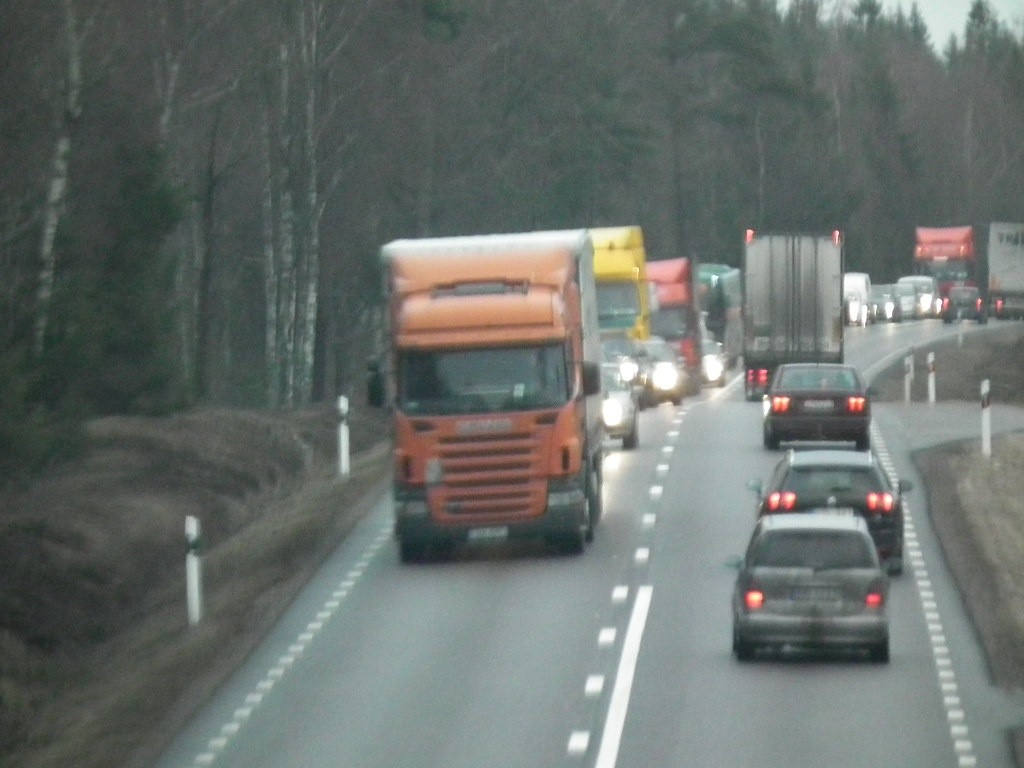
[764,362,872,451]
[727,513,893,664]
[698,336,729,388]
[942,285,988,325]
[644,338,685,407]
[841,271,942,326]
[599,362,642,448]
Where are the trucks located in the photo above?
[911,224,980,302]
[364,230,607,563]
[588,227,741,397]
[988,220,1024,322]
[740,225,845,401]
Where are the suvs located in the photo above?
[749,447,913,579]
